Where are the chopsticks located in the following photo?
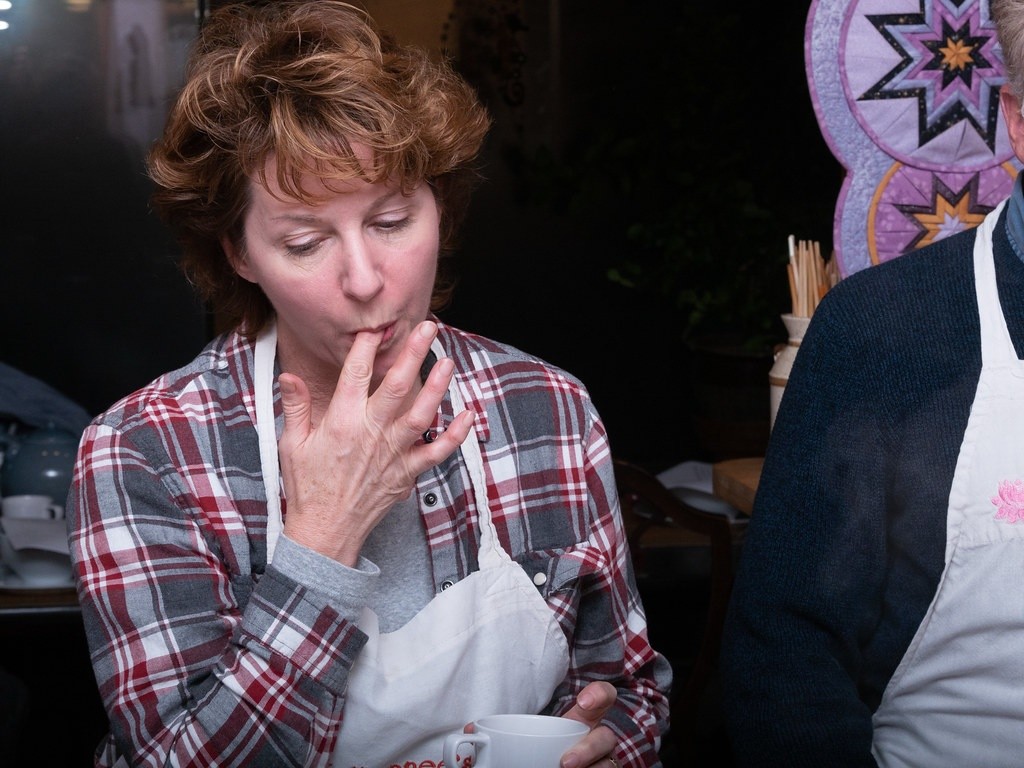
[787,240,840,317]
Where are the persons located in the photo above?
[706,4,1024,768]
[60,0,684,768]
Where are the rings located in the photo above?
[604,751,620,768]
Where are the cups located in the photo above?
[443,714,589,768]
[2,494,62,522]
